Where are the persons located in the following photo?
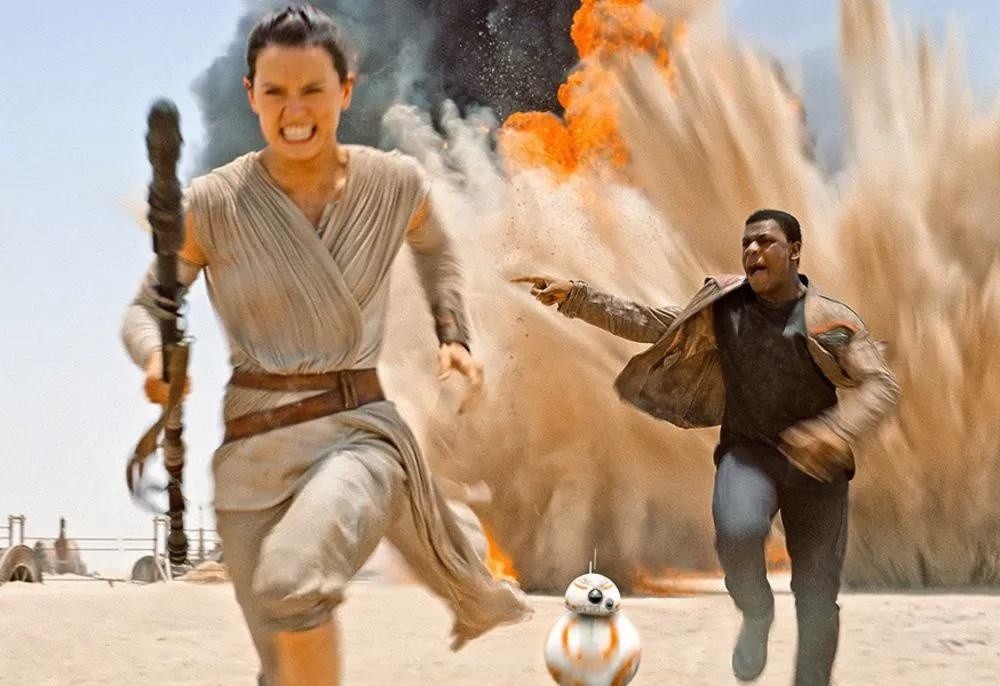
[510,208,903,686]
[121,3,537,685]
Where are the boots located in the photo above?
[725,575,775,681]
